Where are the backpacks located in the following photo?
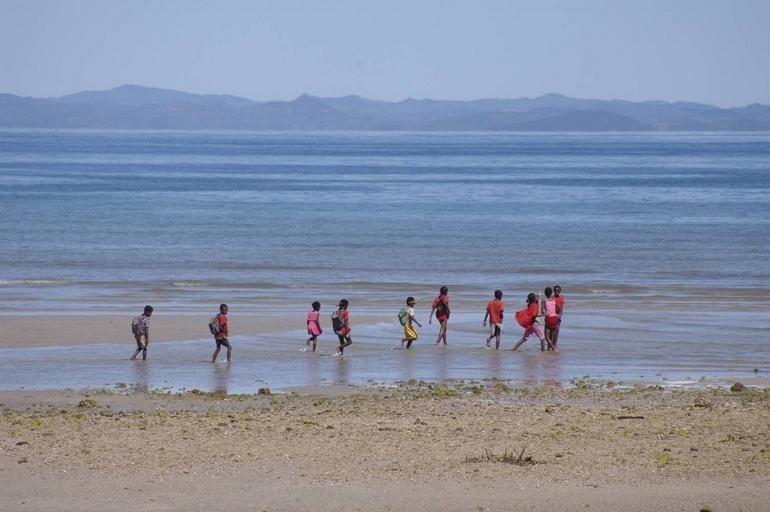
[332,309,345,331]
[398,306,410,326]
[132,312,147,335]
[208,313,221,335]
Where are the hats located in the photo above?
[336,299,348,306]
[407,297,416,305]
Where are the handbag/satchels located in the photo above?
[437,305,446,314]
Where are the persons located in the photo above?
[429,285,451,345]
[130,305,154,360]
[331,299,352,355]
[510,292,547,352]
[549,285,565,349]
[482,290,505,350]
[402,297,422,349]
[541,287,561,353]
[210,304,232,362]
[306,301,323,352]
